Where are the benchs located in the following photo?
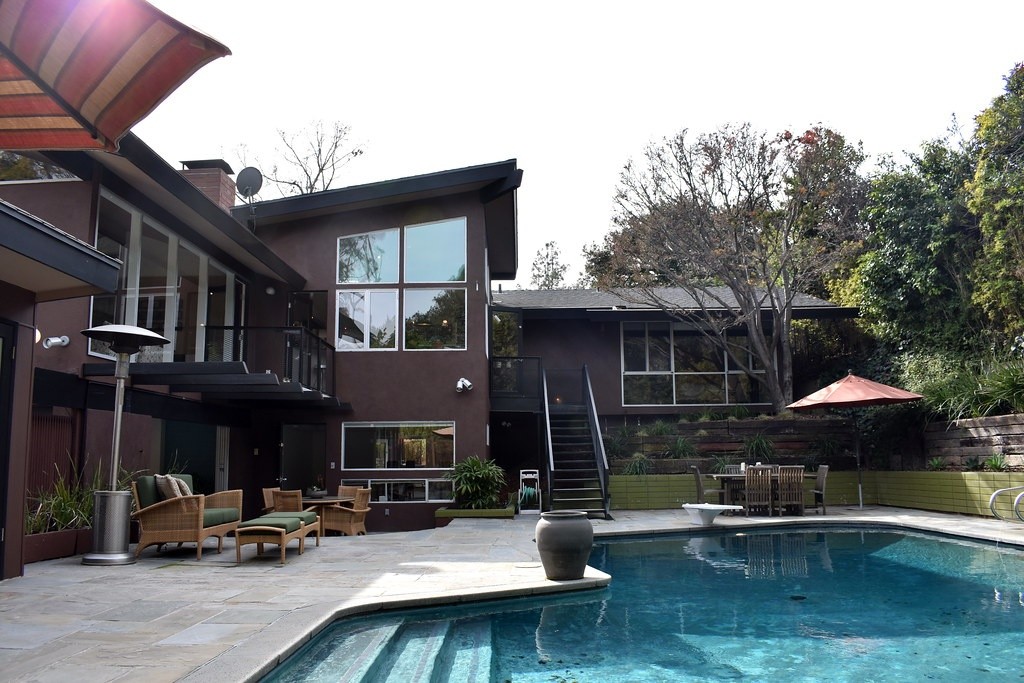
[235,511,321,564]
[127,473,243,561]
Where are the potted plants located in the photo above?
[444,455,508,510]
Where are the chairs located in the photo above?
[261,487,281,515]
[337,486,363,506]
[321,487,372,536]
[272,490,318,512]
[690,465,830,517]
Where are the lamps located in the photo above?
[492,315,501,325]
[463,378,473,391]
[456,379,463,392]
[41,336,70,349]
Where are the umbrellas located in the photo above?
[786,369,924,509]
[0,0,231,154]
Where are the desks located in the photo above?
[280,496,355,506]
[706,473,818,515]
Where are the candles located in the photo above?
[741,463,745,470]
[756,462,761,465]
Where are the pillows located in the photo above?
[171,476,198,510]
[154,474,181,501]
